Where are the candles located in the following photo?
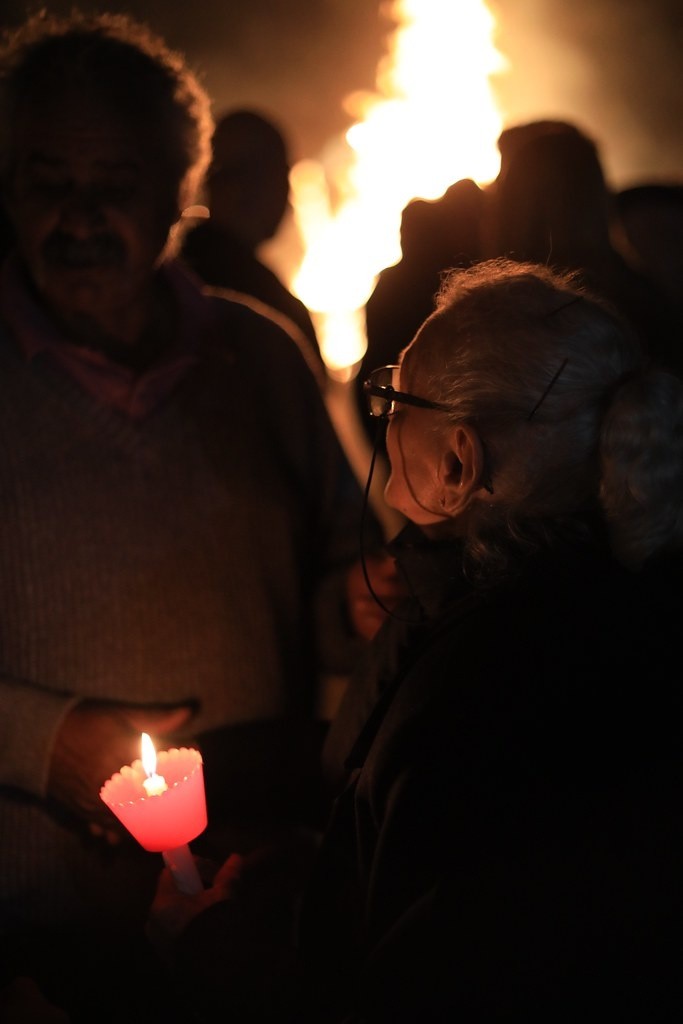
[97,731,207,895]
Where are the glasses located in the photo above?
[362,364,490,477]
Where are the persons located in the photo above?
[296,253,683,939]
[180,108,328,375]
[1,7,388,935]
